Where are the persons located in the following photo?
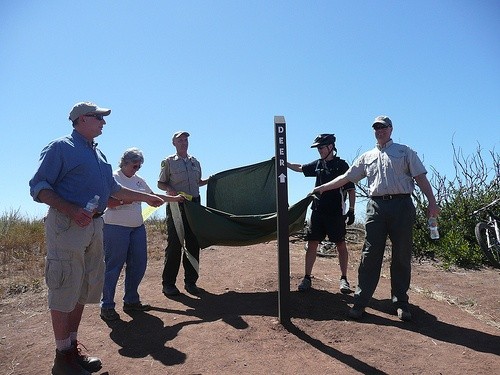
[158,131,208,296]
[101,149,185,321]
[310,116,440,320]
[29,102,163,375]
[286,134,356,295]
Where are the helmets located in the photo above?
[311,134,336,147]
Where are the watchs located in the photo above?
[120,200,124,205]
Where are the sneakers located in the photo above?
[100,308,120,320]
[122,301,150,312]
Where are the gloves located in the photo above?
[345,210,354,225]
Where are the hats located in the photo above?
[172,131,189,145]
[69,101,111,122]
[371,116,392,127]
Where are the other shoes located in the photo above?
[349,305,365,318]
[340,279,351,291]
[185,283,199,294]
[162,285,179,295]
[397,306,412,320]
[51,346,91,375]
[298,279,311,289]
[71,339,103,370]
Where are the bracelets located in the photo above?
[349,208,354,209]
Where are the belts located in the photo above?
[371,194,410,201]
[92,213,105,218]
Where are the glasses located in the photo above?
[374,125,387,130]
[84,113,103,120]
[129,163,141,169]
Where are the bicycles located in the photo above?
[469,199,500,267]
[290,221,367,258]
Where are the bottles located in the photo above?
[429,216,439,239]
[83,194,100,219]
